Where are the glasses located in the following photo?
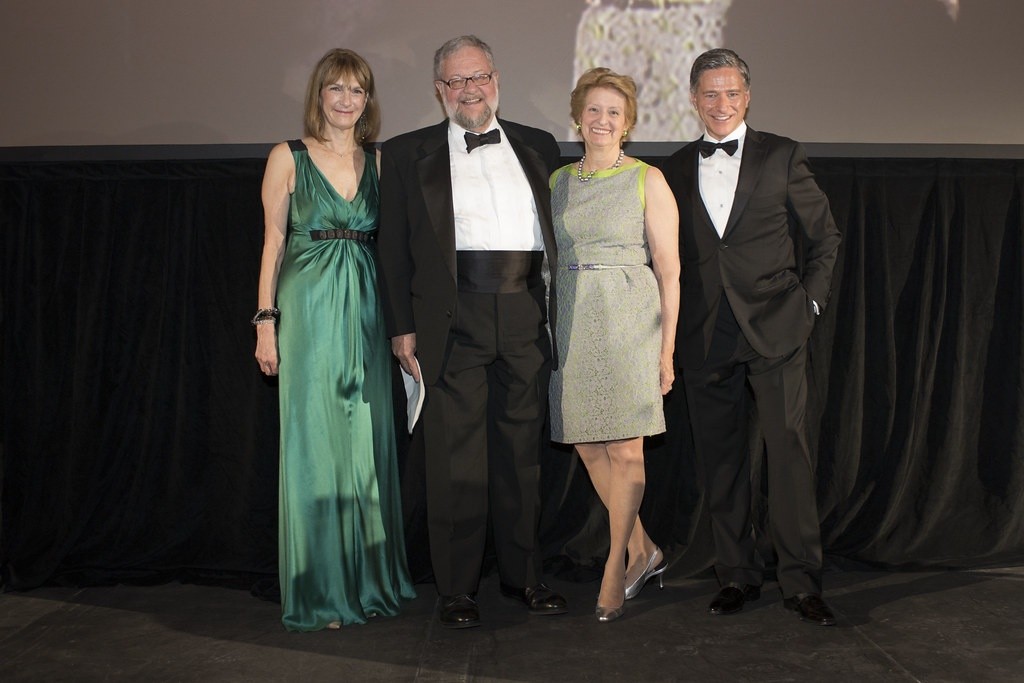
[439,71,494,90]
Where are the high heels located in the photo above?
[624,543,668,600]
[595,592,628,621]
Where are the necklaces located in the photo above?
[578,148,625,182]
[319,141,362,158]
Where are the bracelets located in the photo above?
[251,305,281,325]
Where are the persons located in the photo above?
[378,35,570,628]
[661,48,841,627]
[548,67,681,621]
[251,47,418,633]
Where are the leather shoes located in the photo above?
[786,592,838,626]
[707,581,760,616]
[431,588,481,628]
[503,581,567,614]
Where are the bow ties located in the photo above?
[697,139,739,158]
[464,128,501,154]
